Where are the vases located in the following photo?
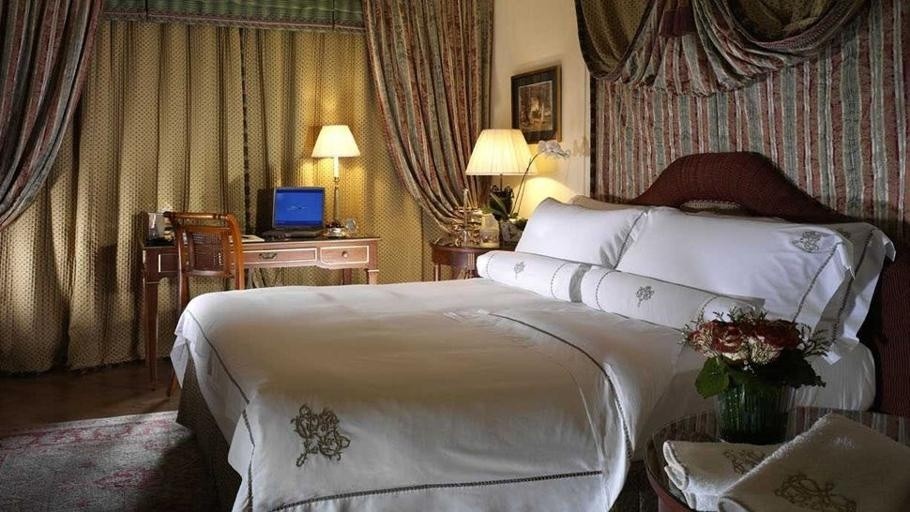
[717,381,791,445]
[499,219,527,243]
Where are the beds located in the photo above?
[168,151,876,512]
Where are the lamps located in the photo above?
[464,127,538,221]
[311,124,361,238]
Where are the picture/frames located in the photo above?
[510,63,563,145]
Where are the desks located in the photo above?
[137,232,382,391]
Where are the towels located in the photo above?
[717,411,910,512]
[660,438,786,512]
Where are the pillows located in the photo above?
[476,249,590,303]
[515,196,649,270]
[814,222,897,366]
[579,264,754,341]
[613,206,856,351]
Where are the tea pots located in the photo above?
[146,212,166,246]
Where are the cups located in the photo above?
[479,229,500,248]
[328,228,342,235]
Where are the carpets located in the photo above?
[0,410,221,512]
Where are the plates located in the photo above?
[323,234,347,238]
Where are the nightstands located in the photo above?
[643,405,909,512]
[429,240,515,281]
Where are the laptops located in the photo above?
[260,186,325,239]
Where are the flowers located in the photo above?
[676,305,832,400]
[480,139,571,220]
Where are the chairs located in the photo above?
[162,209,246,398]
